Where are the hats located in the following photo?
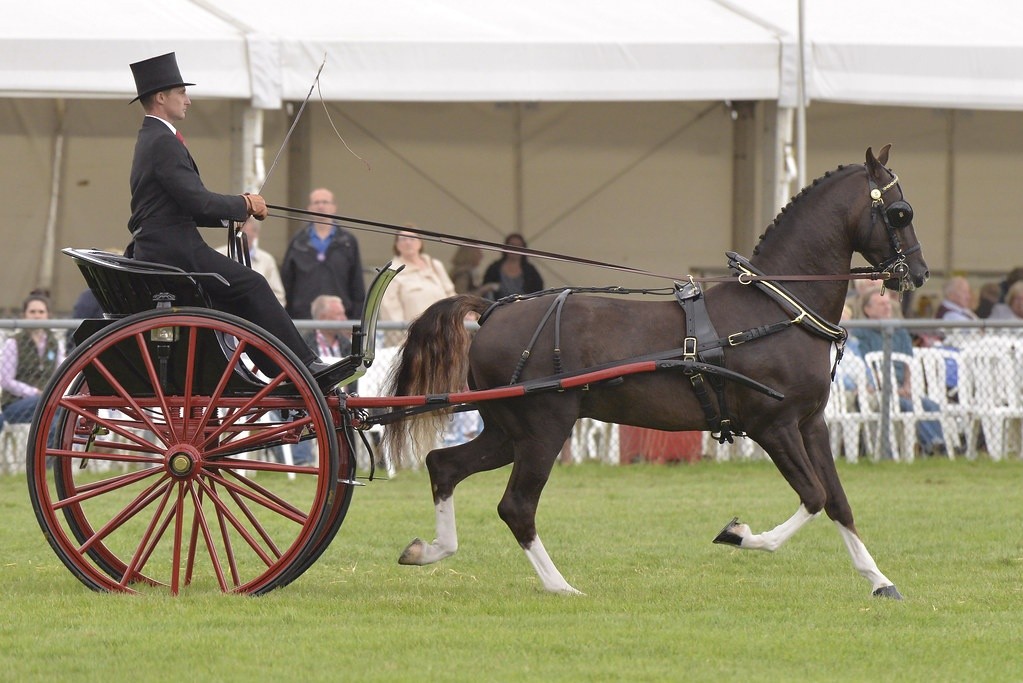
[129,51,196,103]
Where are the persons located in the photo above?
[215,218,287,310]
[379,224,458,347]
[935,276,979,332]
[302,295,352,358]
[853,279,967,456]
[128,51,330,379]
[281,187,365,319]
[975,268,1023,321]
[448,243,483,295]
[832,304,903,448]
[482,234,544,304]
[65,248,125,355]
[0,287,65,467]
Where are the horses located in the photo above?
[369,143,931,602]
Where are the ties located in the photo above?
[176,130,185,146]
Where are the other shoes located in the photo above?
[306,362,330,375]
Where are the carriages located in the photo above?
[26,145,930,601]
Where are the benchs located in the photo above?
[61,245,262,395]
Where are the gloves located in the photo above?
[238,192,268,221]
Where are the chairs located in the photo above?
[714,331,1023,463]
[0,344,623,477]
